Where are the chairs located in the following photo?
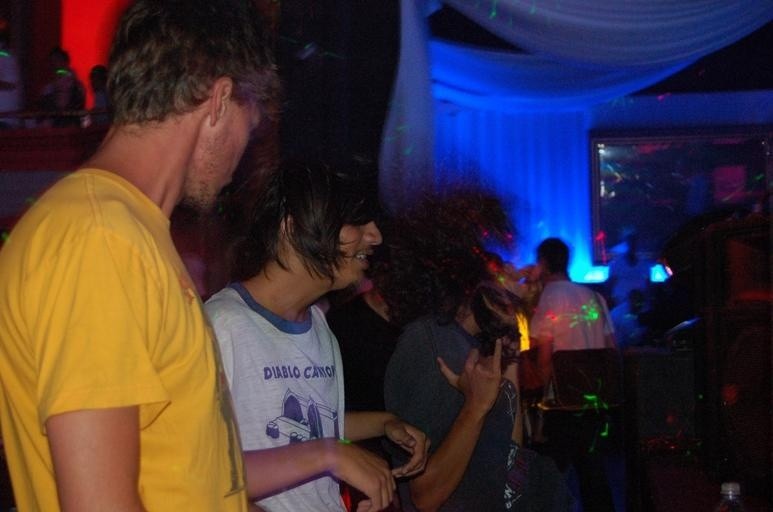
[534,347,623,443]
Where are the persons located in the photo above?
[0,1,773,512]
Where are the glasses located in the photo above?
[345,200,383,225]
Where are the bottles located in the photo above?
[713,480,746,512]
